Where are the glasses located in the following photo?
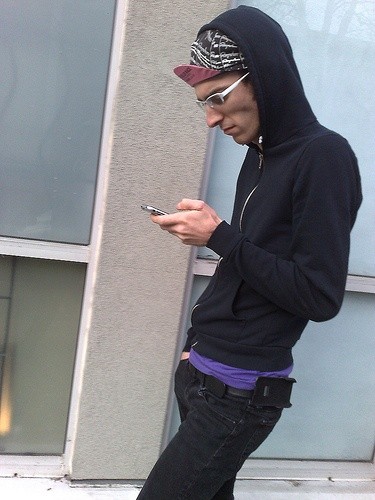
[196,72,250,111]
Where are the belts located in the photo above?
[187,362,254,398]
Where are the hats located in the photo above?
[173,29,248,86]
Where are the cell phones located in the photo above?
[141,203,169,216]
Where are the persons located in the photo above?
[135,5,362,500]
